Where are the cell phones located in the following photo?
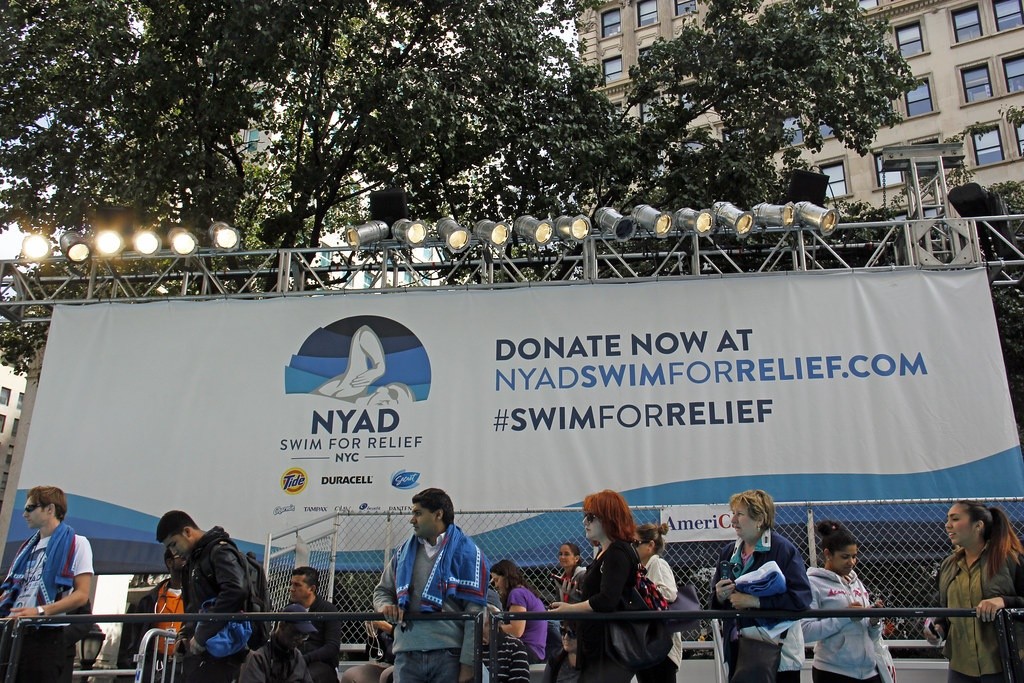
[924,619,943,643]
[550,572,576,587]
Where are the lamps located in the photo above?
[21,199,838,265]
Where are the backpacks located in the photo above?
[200,537,272,651]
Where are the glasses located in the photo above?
[632,539,651,548]
[558,626,578,639]
[584,512,603,523]
[24,504,42,512]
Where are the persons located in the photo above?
[274,566,342,681]
[155,510,254,682]
[704,489,813,683]
[238,603,320,682]
[921,499,1024,683]
[798,519,886,683]
[546,489,684,683]
[374,488,489,683]
[490,559,550,664]
[128,546,189,683]
[1,485,95,682]
[481,589,532,683]
[341,618,395,682]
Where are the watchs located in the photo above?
[37,605,46,617]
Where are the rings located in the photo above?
[722,591,728,596]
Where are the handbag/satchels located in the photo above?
[599,543,673,669]
[667,584,702,633]
[730,637,782,683]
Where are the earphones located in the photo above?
[377,647,384,658]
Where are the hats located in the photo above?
[283,604,319,633]
